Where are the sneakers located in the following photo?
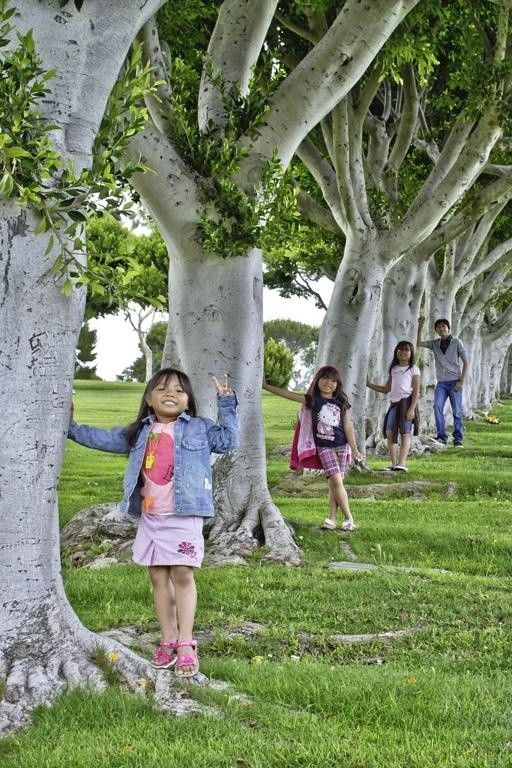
[384,463,408,471]
[455,443,464,448]
[429,437,446,446]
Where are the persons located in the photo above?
[68,369,240,678]
[367,341,420,471]
[416,316,468,448]
[262,365,363,531]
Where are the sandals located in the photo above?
[151,640,177,670]
[341,519,357,531]
[321,519,336,530]
[174,640,199,679]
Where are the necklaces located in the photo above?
[145,422,166,469]
